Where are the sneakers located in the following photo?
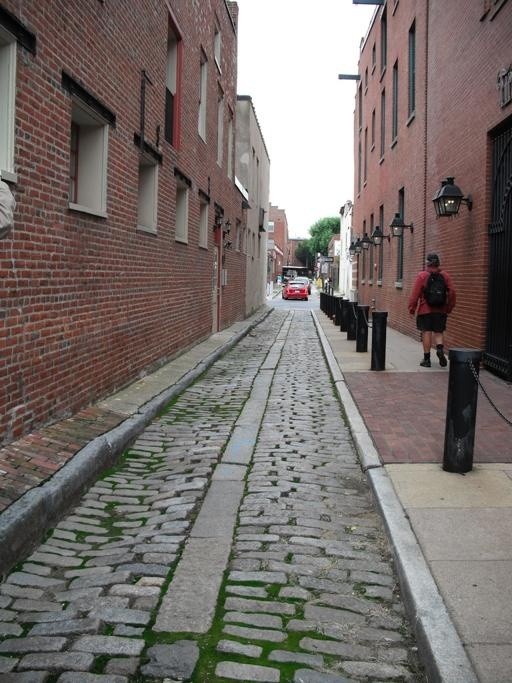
[420,360,430,367]
[436,349,447,366]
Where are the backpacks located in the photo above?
[422,270,447,307]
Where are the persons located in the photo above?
[275,274,282,286]
[407,252,456,367]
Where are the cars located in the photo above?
[283,274,312,295]
[281,280,310,301]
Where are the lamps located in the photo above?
[347,225,390,254]
[431,175,473,216]
[389,212,414,236]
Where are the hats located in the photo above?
[427,254,438,261]
